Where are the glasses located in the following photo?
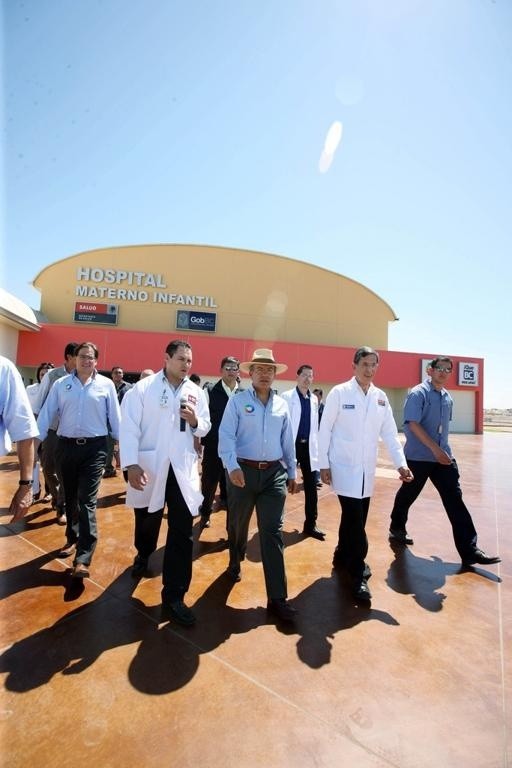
[432,365,453,373]
[222,365,240,372]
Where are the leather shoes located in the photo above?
[161,597,198,628]
[57,541,77,557]
[267,596,301,618]
[71,563,91,579]
[459,551,502,567]
[198,511,213,528]
[131,551,150,575]
[387,522,415,544]
[34,488,68,526]
[303,523,327,537]
[333,559,372,605]
[226,556,243,582]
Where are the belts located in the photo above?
[296,438,309,444]
[57,435,110,446]
[236,456,281,470]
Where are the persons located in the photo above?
[390,357,502,566]
[316,347,414,604]
[1,339,155,579]
[188,357,328,537]
[116,341,211,628]
[216,348,302,617]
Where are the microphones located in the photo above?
[180,396,188,432]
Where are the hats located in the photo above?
[237,347,287,377]
